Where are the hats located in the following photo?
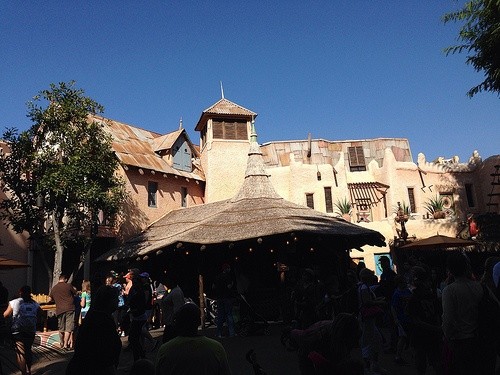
[379,256,388,261]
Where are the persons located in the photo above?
[50,273,76,349]
[122,271,168,351]
[105,273,125,335]
[130,359,154,375]
[156,303,232,375]
[0,281,11,347]
[126,289,146,360]
[3,286,40,375]
[78,279,91,326]
[289,242,500,375]
[64,285,122,375]
[212,264,234,339]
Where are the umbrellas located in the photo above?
[399,235,480,250]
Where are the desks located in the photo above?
[41,304,56,320]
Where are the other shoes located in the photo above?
[121,331,125,337]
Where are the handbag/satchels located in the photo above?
[359,303,385,319]
